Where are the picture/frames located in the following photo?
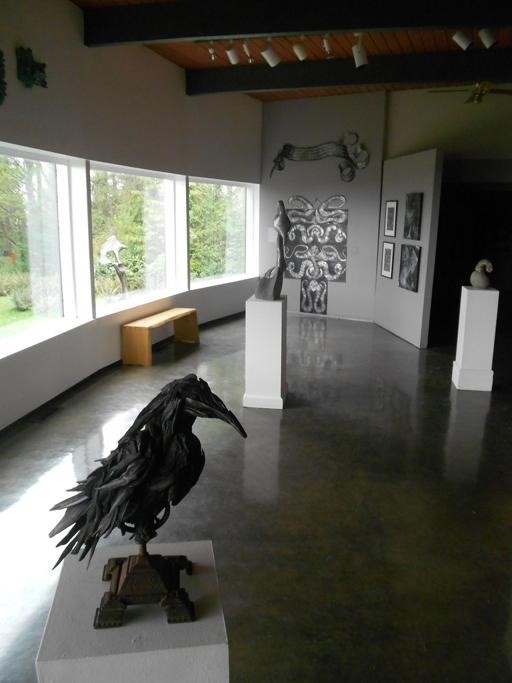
[383,199,398,237]
[380,241,395,278]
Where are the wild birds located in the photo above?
[47,373,249,571]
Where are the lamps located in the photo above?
[207,28,494,69]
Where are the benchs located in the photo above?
[121,307,200,367]
